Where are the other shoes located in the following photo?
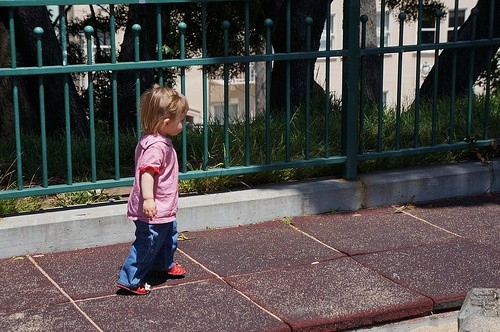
[162,263,186,275]
[116,283,150,295]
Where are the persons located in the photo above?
[117,87,189,294]
